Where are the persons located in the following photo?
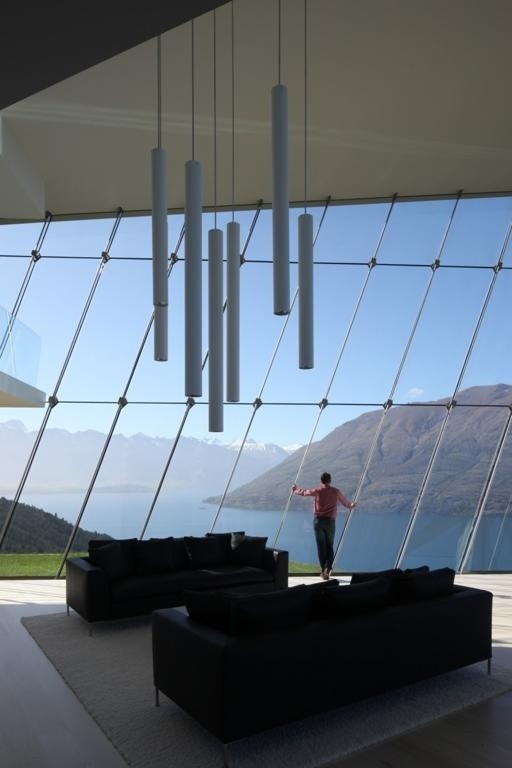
[291,471,358,580]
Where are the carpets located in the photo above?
[20,609,512,768]
